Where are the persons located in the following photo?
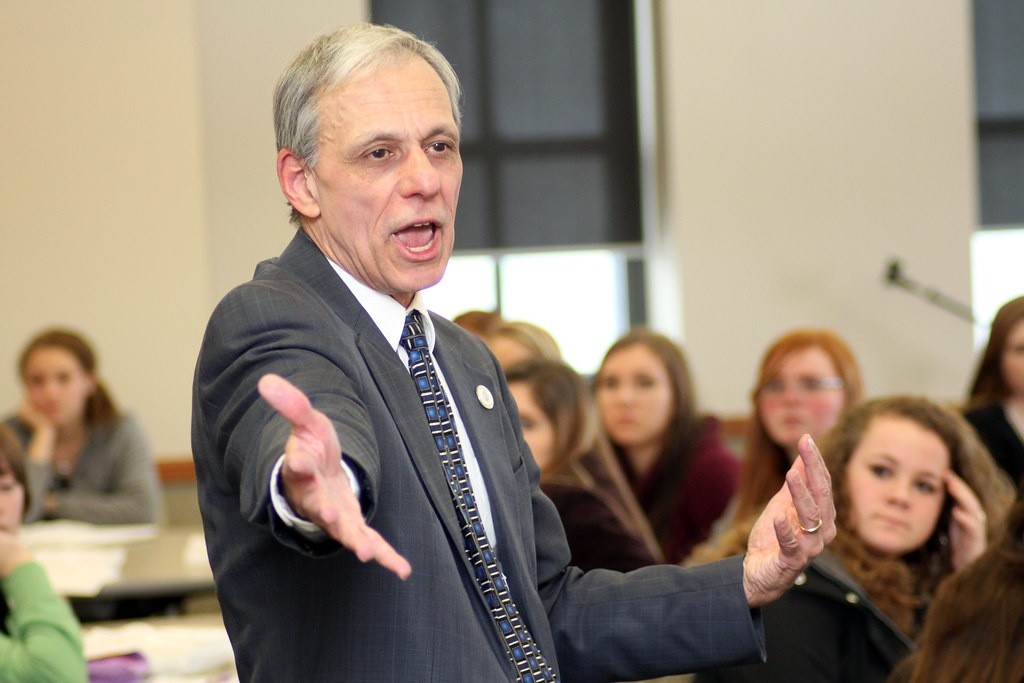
[887,494,1024,683]
[964,298,1024,495]
[0,422,89,683]
[594,332,743,565]
[692,395,1014,683]
[0,329,183,621]
[686,332,863,567]
[191,23,837,683]
[454,311,658,572]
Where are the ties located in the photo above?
[400,309,556,683]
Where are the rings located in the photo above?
[799,519,822,534]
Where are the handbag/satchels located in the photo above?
[88,651,148,683]
